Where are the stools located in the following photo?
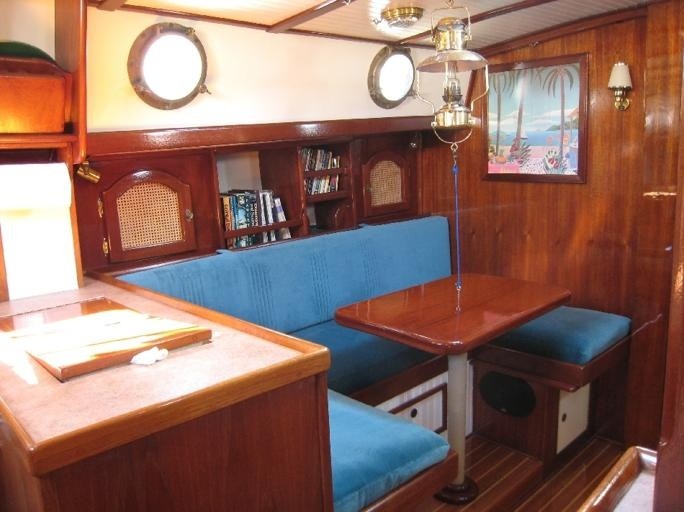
[469,304,629,463]
[327,389,459,512]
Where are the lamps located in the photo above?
[368,43,415,109]
[607,61,632,110]
[416,0,489,152]
[126,23,211,112]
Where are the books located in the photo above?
[219,188,293,251]
[298,146,341,196]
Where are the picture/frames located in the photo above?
[479,50,589,184]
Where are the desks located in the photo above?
[1,279,330,511]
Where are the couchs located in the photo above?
[114,212,474,446]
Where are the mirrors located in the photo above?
[2,159,81,300]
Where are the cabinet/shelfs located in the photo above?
[75,132,418,275]
